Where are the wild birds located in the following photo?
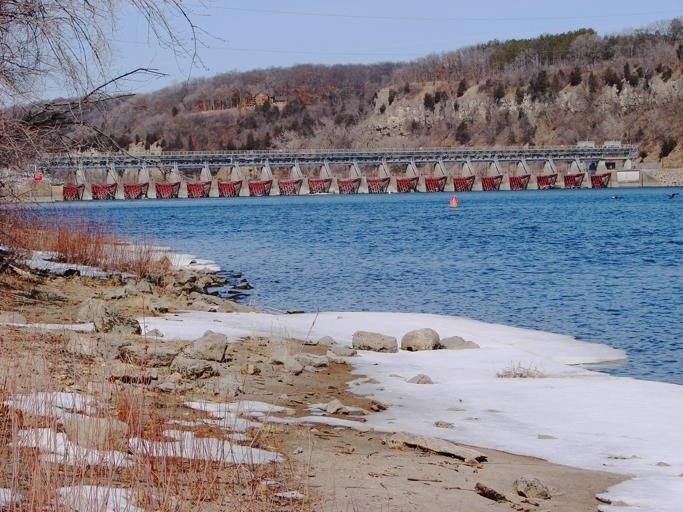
[665,193,680,200]
